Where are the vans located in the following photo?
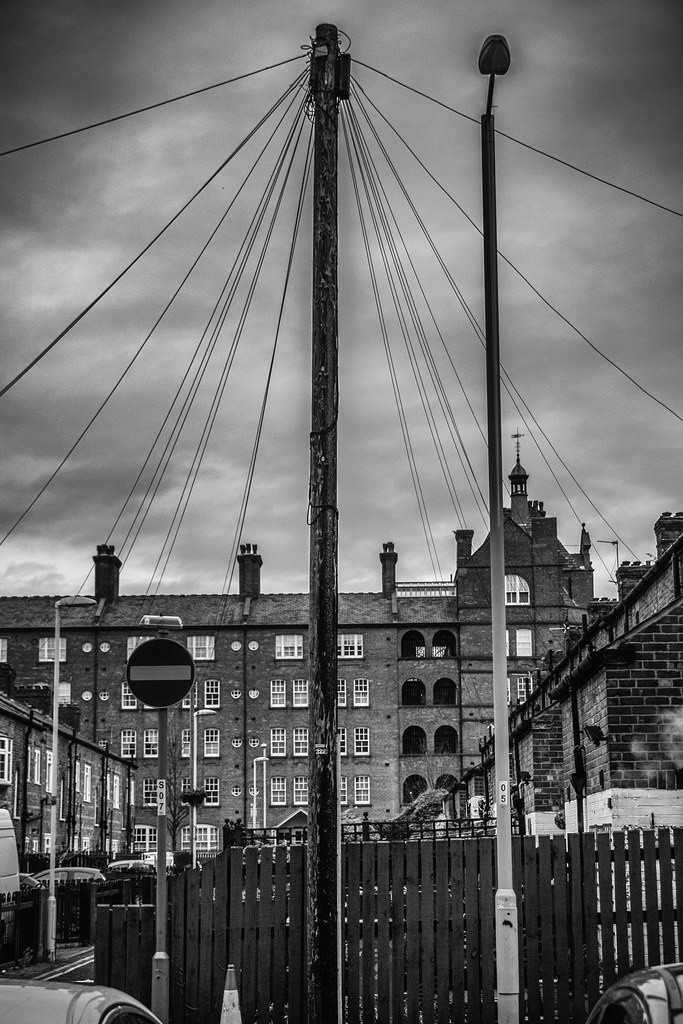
[0,808,21,945]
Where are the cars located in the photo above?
[0,980,160,1023]
[586,962,683,1024]
[19,860,157,902]
[144,856,201,873]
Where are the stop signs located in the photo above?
[125,637,196,707]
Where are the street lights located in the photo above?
[253,757,269,835]
[193,710,218,873]
[46,596,97,961]
[260,743,268,827]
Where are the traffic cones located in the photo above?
[220,965,242,1024]
